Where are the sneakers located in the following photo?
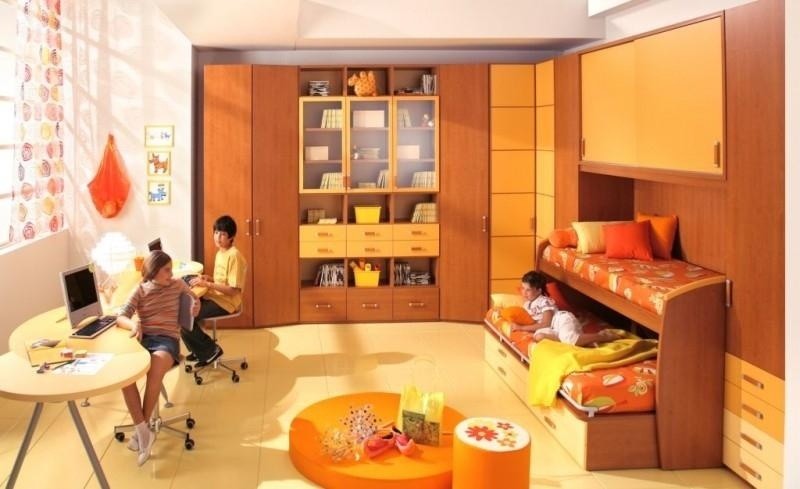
[194,345,224,368]
[137,430,157,467]
[126,432,140,452]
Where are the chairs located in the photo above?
[114,293,196,451]
[183,289,249,386]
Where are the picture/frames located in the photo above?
[147,180,172,206]
[146,151,172,177]
[144,124,175,148]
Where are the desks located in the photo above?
[1,258,204,489]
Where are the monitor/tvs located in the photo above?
[58,262,103,329]
[147,237,163,251]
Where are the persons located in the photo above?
[115,250,201,467]
[512,271,622,348]
[181,216,248,368]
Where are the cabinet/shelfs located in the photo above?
[202,65,299,330]
[723,352,786,489]
[299,65,440,322]
[439,65,490,324]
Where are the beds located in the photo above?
[483,232,725,470]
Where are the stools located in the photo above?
[452,415,531,488]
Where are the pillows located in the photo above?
[602,220,654,262]
[543,282,569,309]
[637,212,677,260]
[501,307,534,324]
[549,229,578,249]
[572,221,636,254]
[492,292,529,307]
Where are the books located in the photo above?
[410,171,435,188]
[397,108,411,128]
[359,147,381,160]
[422,74,437,95]
[317,215,338,225]
[321,109,343,129]
[395,262,429,285]
[358,182,376,188]
[315,264,345,287]
[319,172,344,190]
[376,169,387,188]
[409,203,437,223]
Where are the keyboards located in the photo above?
[70,314,118,340]
[181,272,199,288]
